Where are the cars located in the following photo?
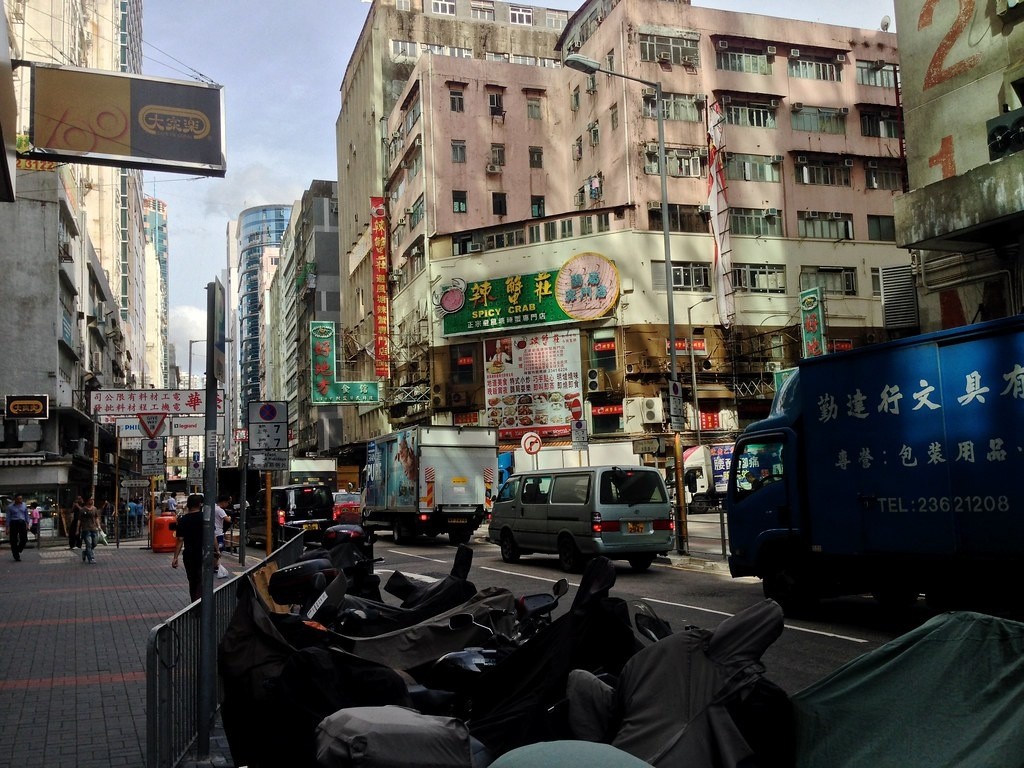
[332,491,363,525]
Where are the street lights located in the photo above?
[185,337,233,496]
[687,295,715,447]
[564,52,690,555]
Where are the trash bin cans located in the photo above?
[151,512,182,553]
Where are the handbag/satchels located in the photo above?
[98,530,108,546]
[216,558,229,579]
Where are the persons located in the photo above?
[6,491,233,614]
[745,472,762,490]
[491,340,513,366]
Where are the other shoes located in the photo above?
[15,557,21,561]
[82,550,86,562]
[90,559,97,564]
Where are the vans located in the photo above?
[486,464,677,571]
[245,483,334,554]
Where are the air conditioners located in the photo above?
[804,211,841,220]
[841,159,878,169]
[587,368,606,392]
[764,208,777,216]
[486,164,502,175]
[646,144,708,157]
[642,398,662,423]
[769,100,803,112]
[765,362,780,373]
[832,54,885,70]
[716,40,728,52]
[838,108,892,117]
[766,46,800,59]
[388,203,420,281]
[657,52,697,65]
[647,200,710,213]
[469,243,482,252]
[392,131,421,168]
[642,88,706,103]
[769,155,808,165]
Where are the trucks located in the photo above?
[361,422,500,544]
[724,314,1024,623]
[661,441,784,516]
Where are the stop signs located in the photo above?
[571,398,584,421]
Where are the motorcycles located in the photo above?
[217,526,800,768]
